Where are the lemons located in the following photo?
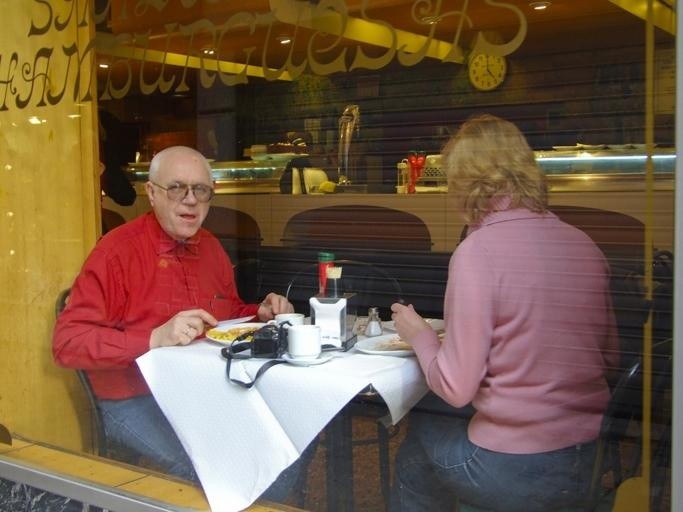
[319,181,335,193]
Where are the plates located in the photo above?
[282,352,333,365]
[354,332,415,357]
[382,318,447,332]
[205,322,270,346]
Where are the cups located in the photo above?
[288,325,322,360]
[267,313,305,328]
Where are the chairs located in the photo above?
[55,285,109,456]
[582,336,672,511]
[284,260,406,512]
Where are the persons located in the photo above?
[390,112,620,512]
[50,143,319,510]
[96,115,136,237]
[279,130,315,194]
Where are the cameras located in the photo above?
[250,319,293,360]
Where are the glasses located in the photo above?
[148,179,214,204]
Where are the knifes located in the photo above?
[204,314,256,329]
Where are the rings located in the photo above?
[184,326,192,335]
